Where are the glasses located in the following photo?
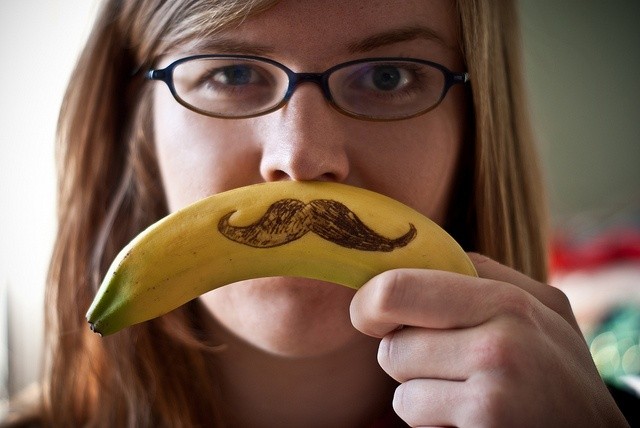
[140,54,471,120]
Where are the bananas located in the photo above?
[84,181,479,335]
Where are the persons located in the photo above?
[1,0,639,427]
